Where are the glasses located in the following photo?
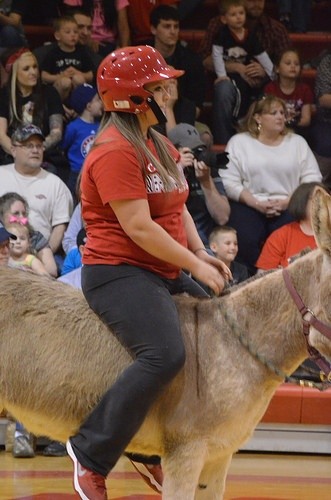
[254,94,266,112]
[11,211,29,218]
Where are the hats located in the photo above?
[71,83,98,114]
[77,229,88,249]
[0,46,32,74]
[168,123,208,151]
[0,221,17,243]
[15,123,45,143]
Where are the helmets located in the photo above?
[97,44,186,114]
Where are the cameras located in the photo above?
[188,147,230,170]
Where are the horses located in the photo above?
[0,183,331,499]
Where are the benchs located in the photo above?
[177,31,331,425]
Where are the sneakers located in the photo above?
[66,438,107,500]
[12,435,35,457]
[131,459,163,495]
[43,440,68,456]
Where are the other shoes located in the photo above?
[299,358,321,374]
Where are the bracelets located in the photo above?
[194,248,209,254]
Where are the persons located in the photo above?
[0,0,331,459]
[65,44,234,500]
